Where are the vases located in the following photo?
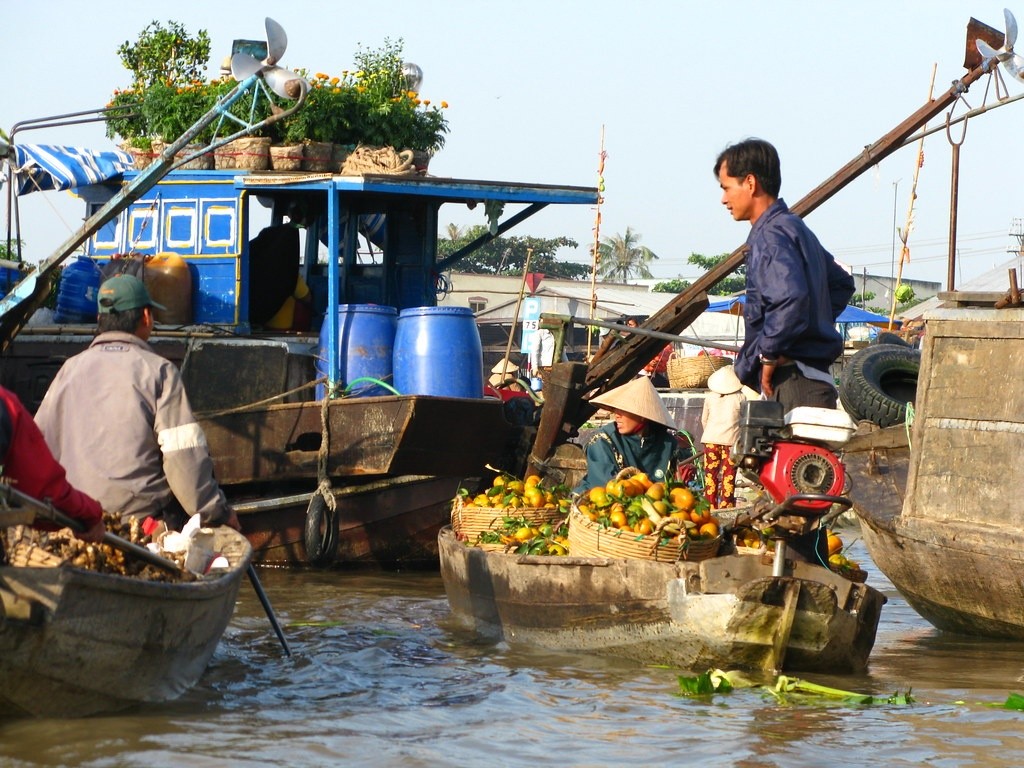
[302,139,334,173]
[162,143,172,151]
[269,143,304,170]
[363,144,384,151]
[334,143,357,174]
[150,141,163,162]
[213,137,236,168]
[235,137,272,169]
[412,150,433,178]
[126,147,152,169]
[174,145,214,170]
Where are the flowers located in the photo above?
[98,17,451,156]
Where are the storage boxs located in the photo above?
[784,405,858,452]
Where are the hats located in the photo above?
[97,273,169,313]
[588,376,679,432]
[707,365,743,395]
[492,358,519,375]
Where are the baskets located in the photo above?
[667,353,733,389]
[451,492,572,554]
[566,466,724,564]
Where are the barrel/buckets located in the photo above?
[52,255,113,325]
[391,304,485,400]
[314,304,399,402]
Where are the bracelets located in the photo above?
[759,354,777,365]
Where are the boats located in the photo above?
[837,314,1024,643]
[0,499,255,732]
[436,525,888,680]
[190,396,510,572]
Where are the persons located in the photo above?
[0,385,105,544]
[627,316,642,328]
[713,136,856,415]
[33,273,241,533]
[700,366,746,509]
[488,358,521,392]
[575,376,692,494]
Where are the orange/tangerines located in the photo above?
[455,474,572,557]
[735,525,861,571]
[576,472,720,543]
